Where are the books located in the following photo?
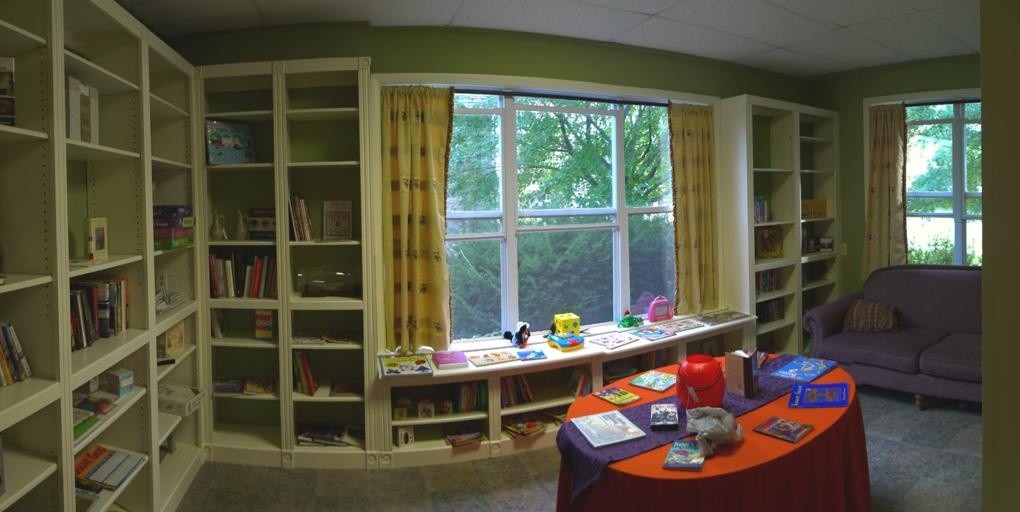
[754,195,785,321]
[73,408,146,491]
[288,193,353,240]
[209,209,278,397]
[85,216,108,261]
[69,277,129,353]
[292,349,316,396]
[0,321,32,387]
[297,417,364,447]
[801,198,826,218]
[65,76,99,145]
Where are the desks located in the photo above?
[566,350,860,512]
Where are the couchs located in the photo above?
[803,261,985,416]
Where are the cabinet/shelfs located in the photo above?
[713,94,841,357]
[0,0,206,510]
[190,54,379,470]
[379,315,756,470]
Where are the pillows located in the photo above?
[844,298,903,338]
